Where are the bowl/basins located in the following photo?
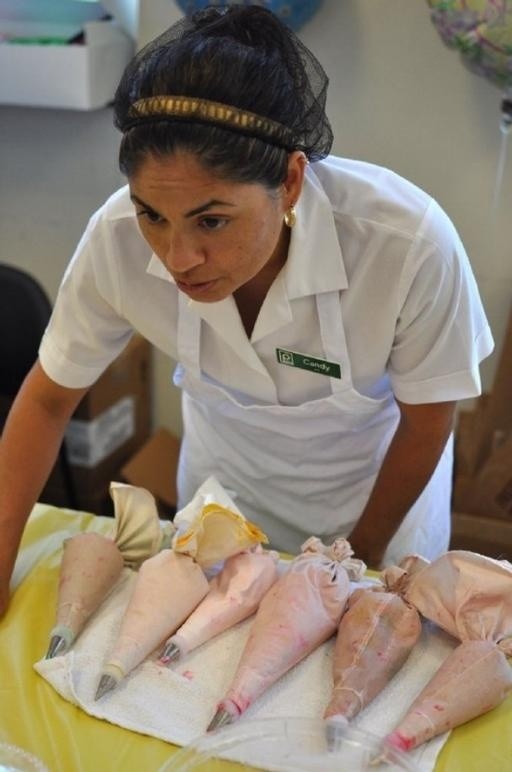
[154,716,430,772]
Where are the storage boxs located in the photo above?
[122,424,181,510]
[34,334,150,518]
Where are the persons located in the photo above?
[0,1,495,623]
